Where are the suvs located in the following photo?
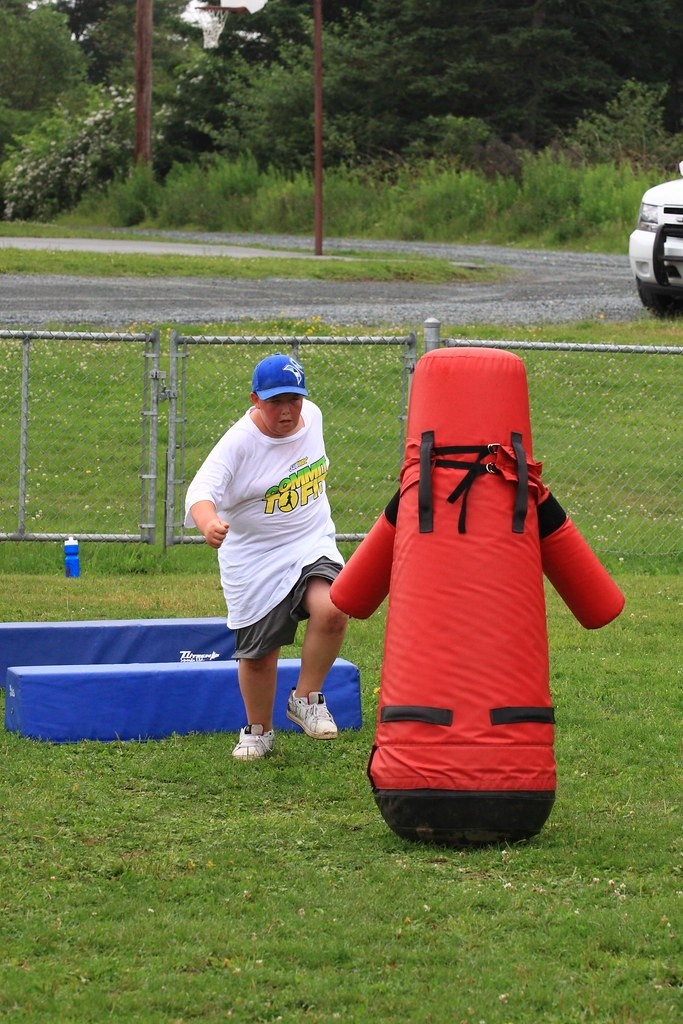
[629,160,683,318]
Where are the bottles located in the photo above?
[64,537,80,579]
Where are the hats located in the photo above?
[252,353,309,401]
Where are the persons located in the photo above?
[186,349,349,765]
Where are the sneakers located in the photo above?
[286,687,338,739]
[231,723,275,762]
